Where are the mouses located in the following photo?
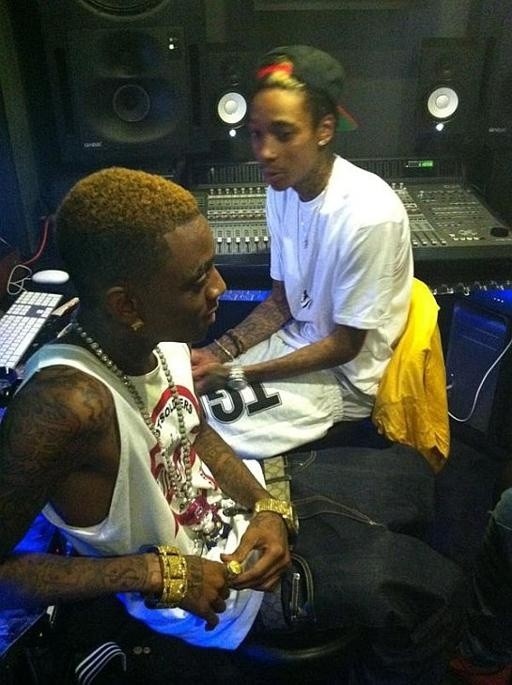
[32,270,69,284]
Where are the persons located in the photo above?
[191,40,416,466]
[0,163,475,685]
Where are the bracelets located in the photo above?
[137,539,190,612]
[212,339,236,362]
[225,329,245,358]
[226,366,248,392]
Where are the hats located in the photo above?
[253,43,359,135]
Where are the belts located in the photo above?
[256,455,292,633]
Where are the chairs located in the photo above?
[285,278,441,453]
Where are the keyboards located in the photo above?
[0,291,64,374]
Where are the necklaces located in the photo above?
[69,302,196,507]
[284,179,330,310]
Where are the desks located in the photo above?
[0,343,86,684]
[212,269,509,313]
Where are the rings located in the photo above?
[223,559,240,586]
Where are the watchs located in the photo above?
[248,495,300,538]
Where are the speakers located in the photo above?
[415,37,489,157]
[42,0,205,175]
[445,289,512,463]
[189,43,256,162]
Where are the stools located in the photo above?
[230,625,372,685]
